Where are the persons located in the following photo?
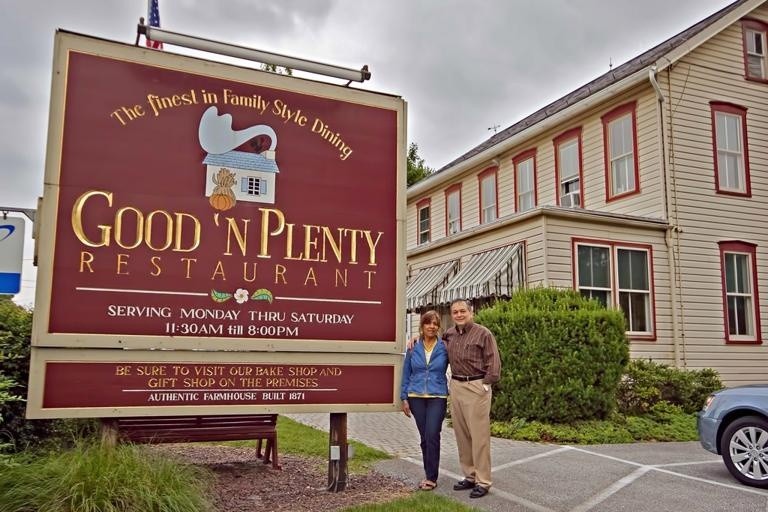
[406,298,502,498]
[400,310,447,491]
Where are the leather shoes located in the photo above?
[469,485,488,499]
[453,478,476,490]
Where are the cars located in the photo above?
[696,381,768,489]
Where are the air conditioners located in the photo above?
[558,190,581,210]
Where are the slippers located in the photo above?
[418,478,438,491]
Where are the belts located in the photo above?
[451,374,485,382]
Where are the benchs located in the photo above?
[95,410,285,473]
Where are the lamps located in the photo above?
[132,16,372,88]
